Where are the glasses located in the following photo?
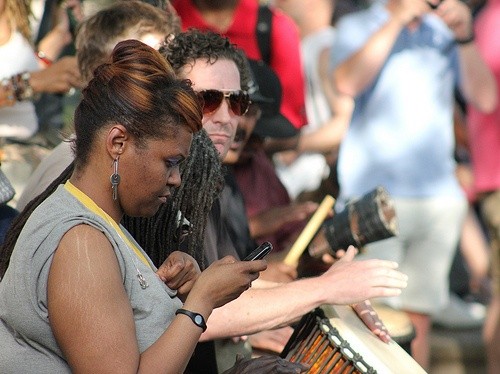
[196,88,254,118]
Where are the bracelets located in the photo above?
[33,47,54,66]
[1,69,34,106]
[455,34,474,44]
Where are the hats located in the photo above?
[244,59,298,139]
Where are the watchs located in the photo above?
[175,309,207,331]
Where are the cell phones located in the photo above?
[244,242,273,263]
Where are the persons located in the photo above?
[1,40,268,374]
[1,0,500,374]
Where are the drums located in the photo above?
[280,304,428,374]
[373,304,418,359]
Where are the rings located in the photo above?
[248,284,251,287]
[60,2,67,9]
